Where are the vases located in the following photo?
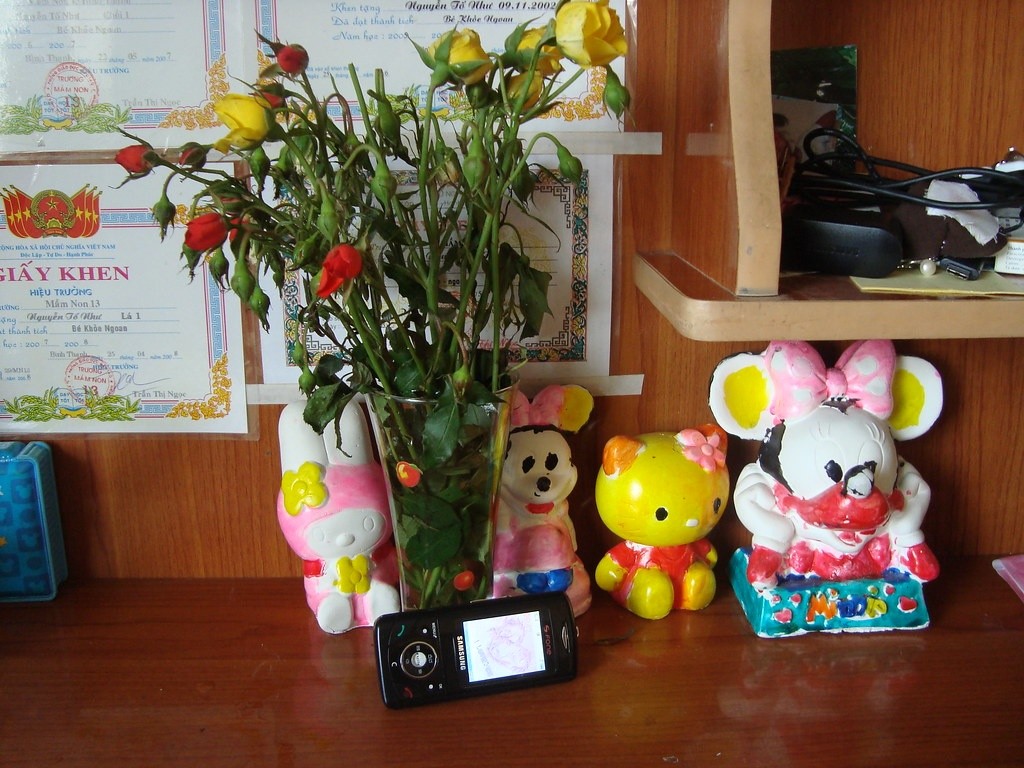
[365,365,522,609]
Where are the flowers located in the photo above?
[99,0,634,609]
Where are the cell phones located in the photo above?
[375,590,577,708]
